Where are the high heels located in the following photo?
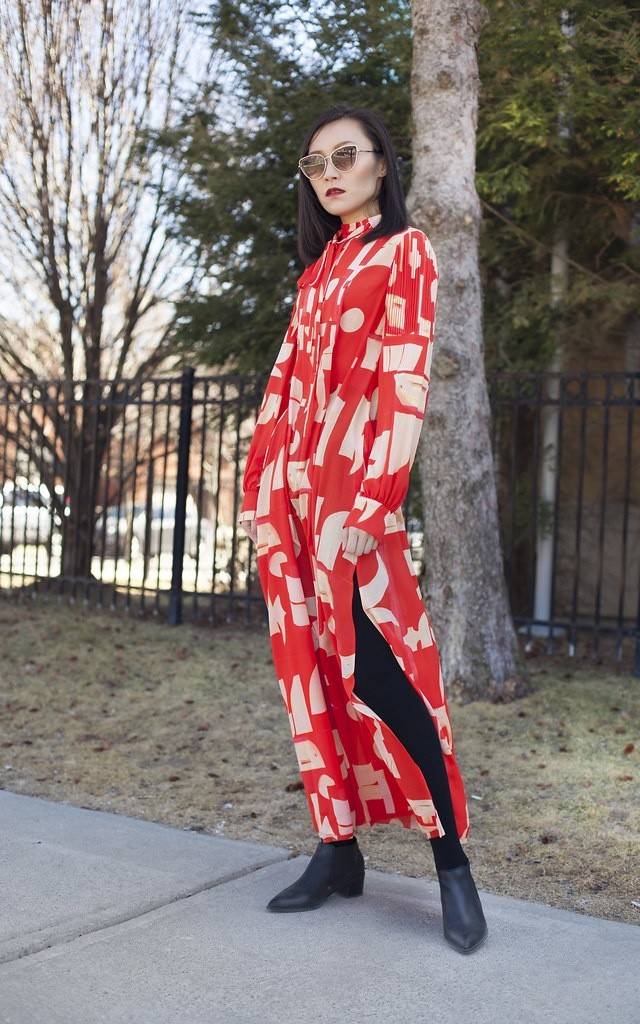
[266,836,365,912]
[437,859,487,955]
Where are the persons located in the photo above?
[240,111,489,952]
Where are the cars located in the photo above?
[91,486,206,564]
[3,479,61,559]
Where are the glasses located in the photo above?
[298,145,375,180]
